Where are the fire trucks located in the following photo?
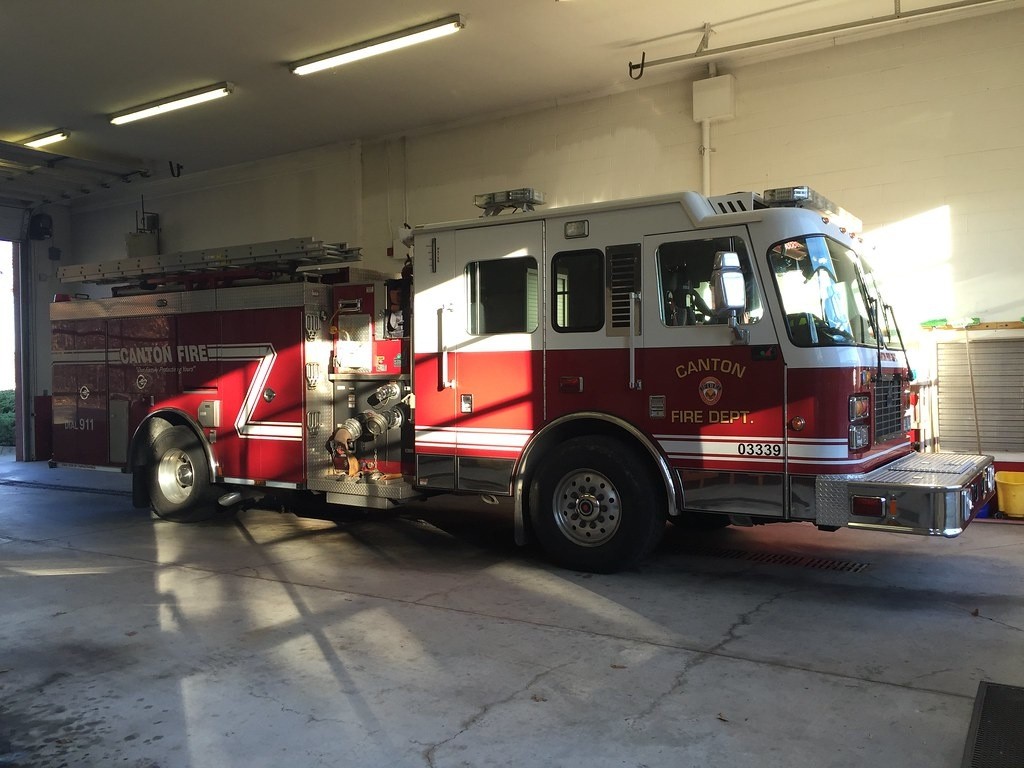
[45,182,1000,576]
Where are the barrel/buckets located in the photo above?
[995,471,1024,518]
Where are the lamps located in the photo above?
[106,81,234,124]
[22,129,71,148]
[288,13,465,76]
